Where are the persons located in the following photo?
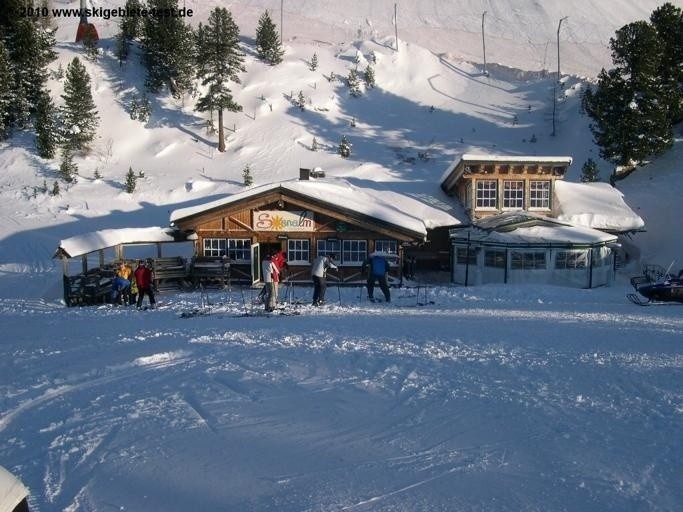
[114,260,131,302]
[261,253,280,311]
[257,253,287,303]
[133,260,158,310]
[110,275,132,306]
[360,251,392,303]
[124,259,140,305]
[308,254,339,306]
[143,257,156,290]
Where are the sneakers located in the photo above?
[151,302,156,309]
[312,300,318,305]
[386,294,390,302]
[137,307,142,310]
[367,296,374,299]
[318,299,324,304]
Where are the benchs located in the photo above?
[64,255,231,307]
